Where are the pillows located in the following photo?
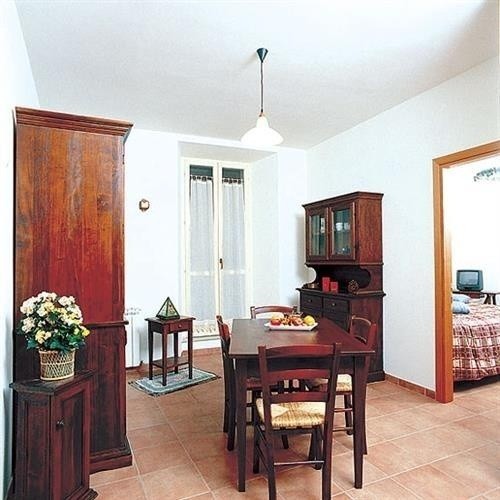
[452,293,472,313]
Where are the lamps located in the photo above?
[241,47,287,147]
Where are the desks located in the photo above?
[452,291,500,305]
[145,315,196,386]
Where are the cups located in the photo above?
[336,223,342,231]
[330,282,339,292]
[344,223,349,230]
[322,277,330,291]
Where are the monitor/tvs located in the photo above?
[457,270,483,292]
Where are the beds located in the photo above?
[452,302,500,381]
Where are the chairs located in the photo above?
[306,315,377,460]
[216,315,290,452]
[250,305,298,423]
[250,341,342,499]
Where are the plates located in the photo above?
[264,322,319,331]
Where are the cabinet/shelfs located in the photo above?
[7,371,100,500]
[12,106,139,472]
[295,287,385,383]
[301,191,384,265]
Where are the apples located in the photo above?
[270,314,315,326]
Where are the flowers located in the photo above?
[14,291,90,353]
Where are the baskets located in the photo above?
[38,347,75,381]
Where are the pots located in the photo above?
[308,282,321,289]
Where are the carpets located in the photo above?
[128,365,221,398]
